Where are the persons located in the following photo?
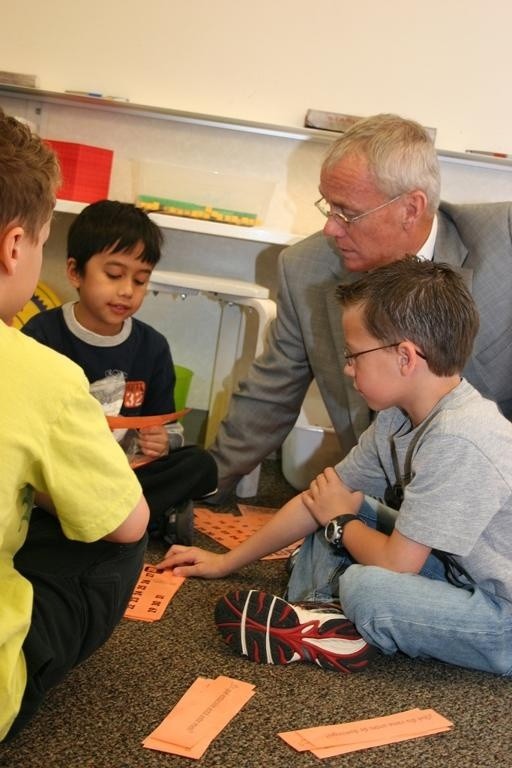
[198,112,511,506]
[154,251,512,676]
[20,198,219,547]
[0,108,150,746]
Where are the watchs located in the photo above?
[325,514,367,555]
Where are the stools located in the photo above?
[129,266,279,505]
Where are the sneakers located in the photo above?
[210,588,377,677]
[155,495,196,553]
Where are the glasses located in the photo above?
[342,342,429,369]
[313,192,408,227]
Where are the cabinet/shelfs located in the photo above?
[0,81,512,278]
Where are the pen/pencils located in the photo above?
[466,150,512,159]
[65,91,129,103]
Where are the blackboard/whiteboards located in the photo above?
[0,0,512,171]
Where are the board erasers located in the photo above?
[305,108,436,146]
[0,71,41,88]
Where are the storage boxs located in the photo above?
[232,447,281,500]
[38,136,115,206]
[280,393,347,492]
[125,154,273,231]
